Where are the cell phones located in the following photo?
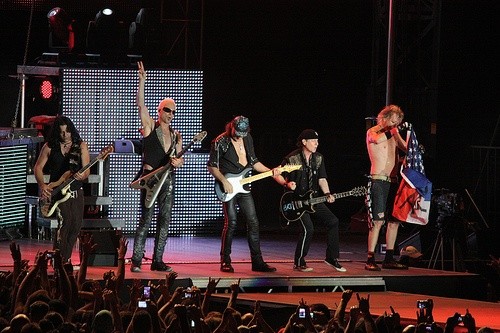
[298,306,306,319]
[143,286,151,298]
[457,315,465,322]
[185,293,193,298]
[137,300,148,308]
[310,312,314,319]
[191,320,195,327]
[47,252,54,258]
[417,300,428,308]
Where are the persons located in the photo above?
[364,104,413,270]
[130,61,187,272]
[207,116,281,272]
[272,128,346,272]
[0,233,494,333]
[33,115,90,266]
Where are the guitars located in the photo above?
[281,186,368,222]
[215,163,302,203]
[38,145,114,217]
[129,131,208,208]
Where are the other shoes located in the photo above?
[365,258,381,271]
[150,261,172,272]
[220,262,234,272]
[293,263,313,272]
[252,262,276,273]
[382,257,409,270]
[130,265,142,272]
[324,258,347,272]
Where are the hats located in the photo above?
[232,115,249,136]
[301,129,319,140]
[400,246,423,258]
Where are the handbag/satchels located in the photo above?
[391,167,432,225]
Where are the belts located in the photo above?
[370,175,397,184]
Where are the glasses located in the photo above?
[163,107,176,115]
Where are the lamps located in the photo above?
[84,7,124,53]
[122,8,164,57]
[38,78,62,115]
[47,7,76,52]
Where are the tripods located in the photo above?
[427,223,462,272]
